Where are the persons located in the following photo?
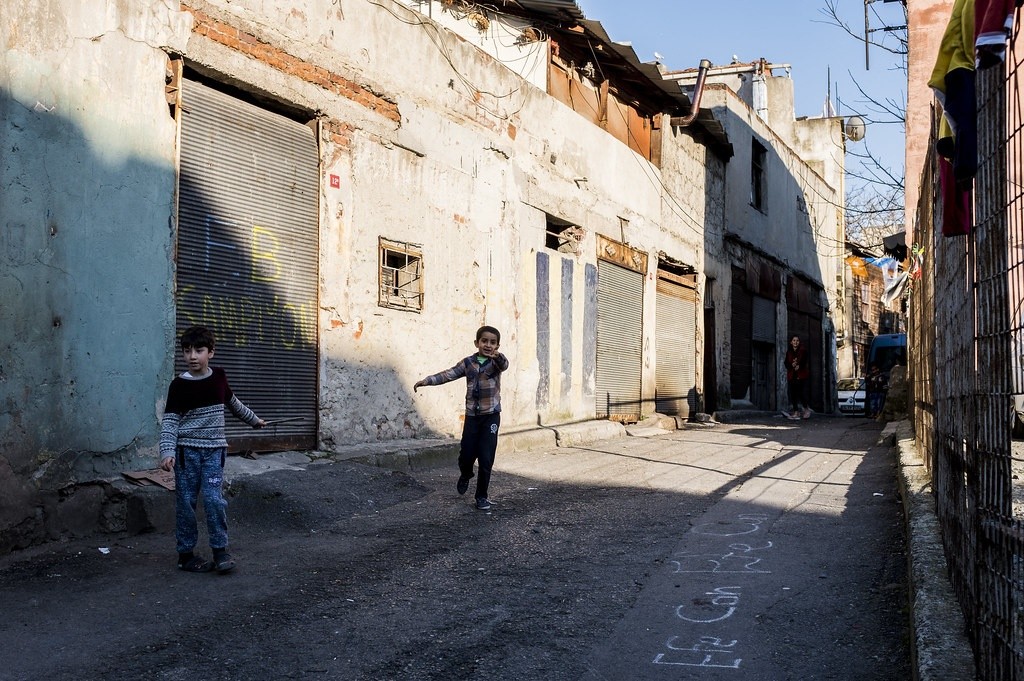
[784,335,812,420]
[159,326,267,573]
[413,326,509,510]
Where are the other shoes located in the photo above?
[788,414,800,420]
[457,472,474,495]
[177,556,217,573]
[476,496,490,509]
[213,553,236,572]
[803,410,812,418]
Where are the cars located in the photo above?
[837,377,866,414]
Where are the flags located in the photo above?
[846,254,911,309]
